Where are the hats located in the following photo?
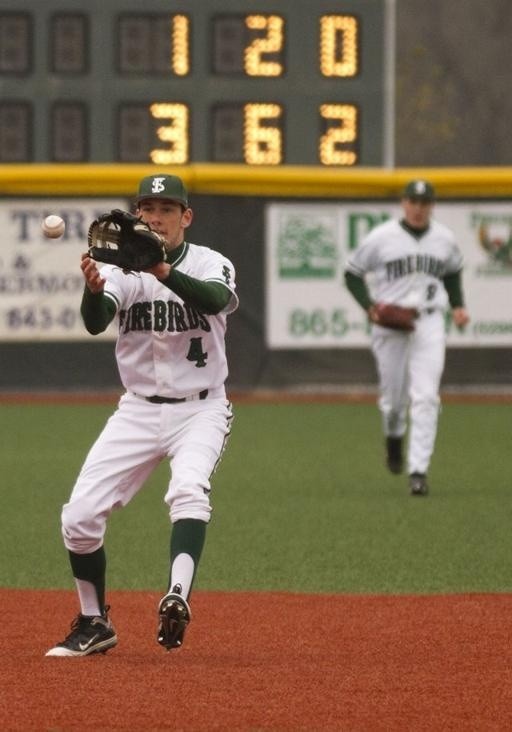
[401,177,437,203]
[133,172,189,209]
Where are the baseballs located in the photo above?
[42,215,65,238]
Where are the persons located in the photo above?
[42,172,241,660]
[342,178,470,499]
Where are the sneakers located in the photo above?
[154,583,193,650]
[384,434,406,476]
[409,471,430,497]
[43,604,119,658]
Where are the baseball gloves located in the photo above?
[88,208,168,276]
[368,302,420,332]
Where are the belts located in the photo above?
[413,307,436,319]
[129,387,211,405]
